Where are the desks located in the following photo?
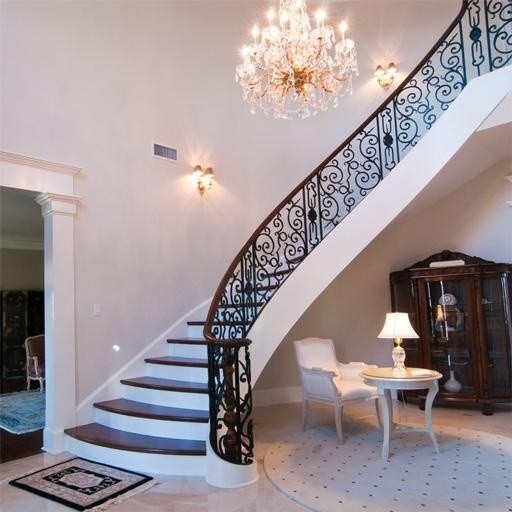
[359,365,443,464]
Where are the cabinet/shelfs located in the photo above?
[386,246,511,417]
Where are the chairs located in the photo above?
[291,335,386,443]
[22,333,46,391]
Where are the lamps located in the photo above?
[374,61,397,95]
[191,162,216,196]
[376,310,424,370]
[225,0,361,123]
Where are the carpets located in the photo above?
[261,415,510,511]
[1,451,165,512]
[0,387,49,436]
